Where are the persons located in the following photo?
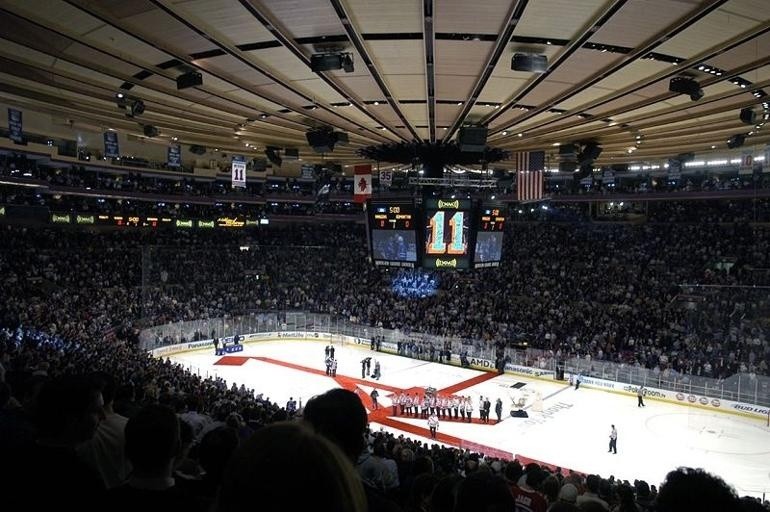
[636,385,647,407]
[0,148,768,379]
[609,425,618,454]
[387,433,769,511]
[0,373,402,509]
[569,373,582,389]
[393,391,503,438]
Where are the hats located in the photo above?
[402,448,413,460]
[492,461,501,471]
[558,484,577,502]
